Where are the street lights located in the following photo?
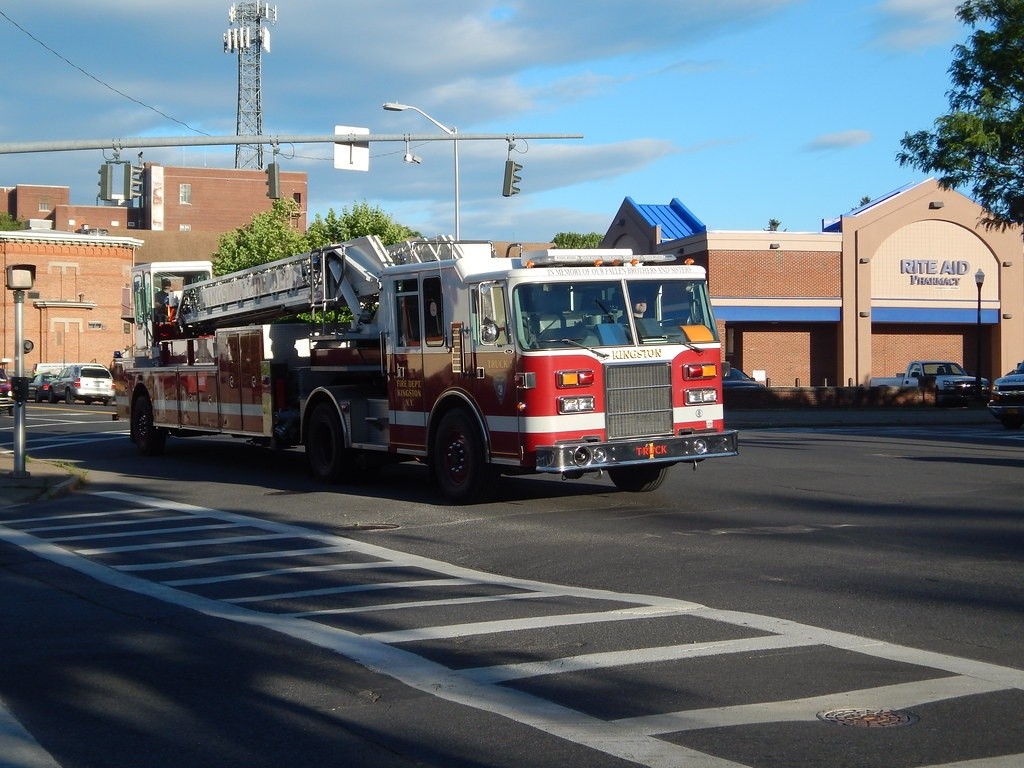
[974,268,985,407]
[383,102,458,240]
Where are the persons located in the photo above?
[617,293,647,324]
[155,278,178,326]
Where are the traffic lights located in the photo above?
[98,165,112,200]
[502,160,522,197]
[265,163,279,200]
[124,163,143,200]
[6,262,35,290]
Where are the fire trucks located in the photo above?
[111,234,740,506]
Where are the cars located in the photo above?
[27,372,56,402]
[722,367,766,390]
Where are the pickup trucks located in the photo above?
[870,361,990,396]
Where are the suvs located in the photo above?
[987,360,1024,422]
[0,369,14,416]
[49,364,115,404]
